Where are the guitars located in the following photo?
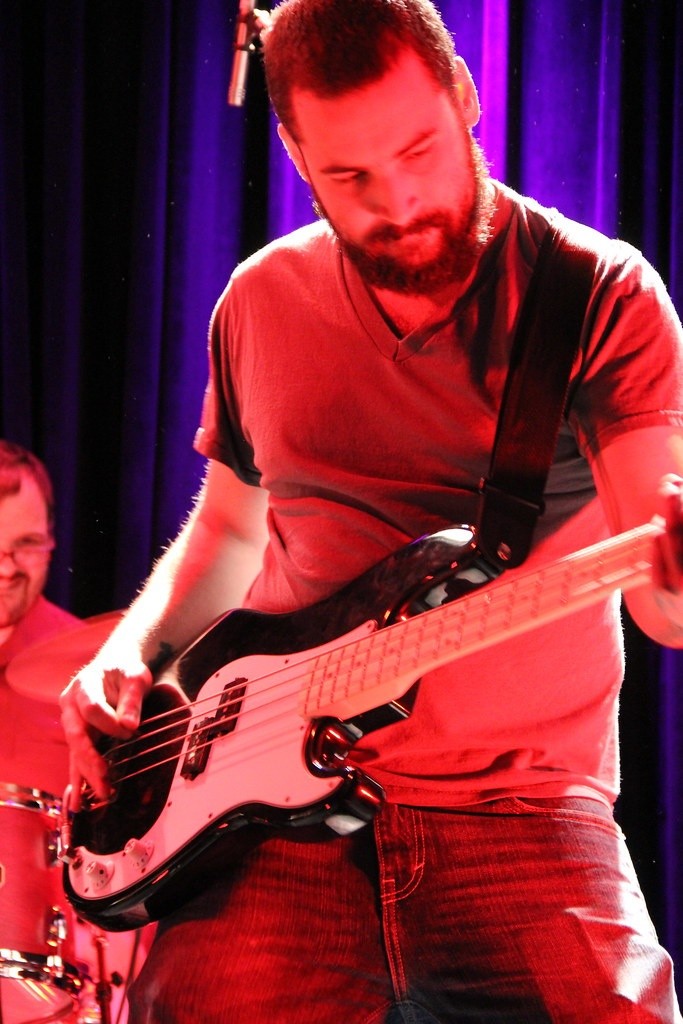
[61,518,681,931]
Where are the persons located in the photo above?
[55,0,683,1024]
[0,440,152,1024]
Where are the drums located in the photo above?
[0,781,87,1023]
